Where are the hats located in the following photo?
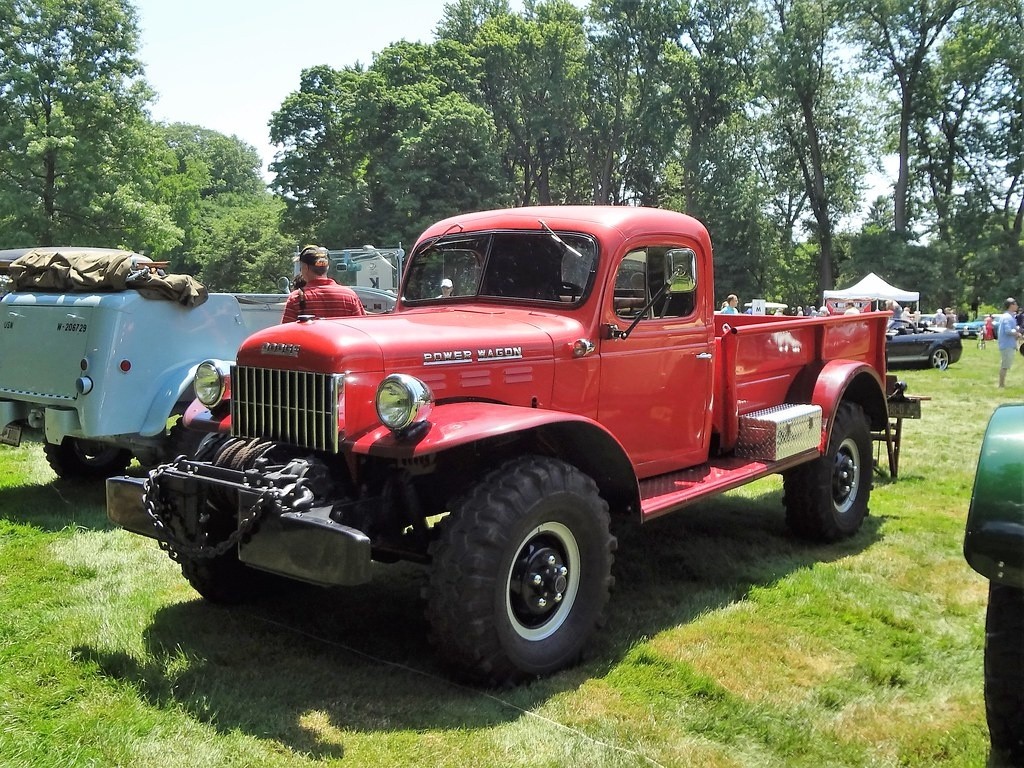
[299,245,333,267]
[1004,297,1016,307]
[440,278,453,288]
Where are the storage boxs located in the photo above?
[735,403,822,461]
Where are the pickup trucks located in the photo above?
[106,204,894,690]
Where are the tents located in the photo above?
[823,273,919,328]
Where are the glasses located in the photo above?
[441,286,451,289]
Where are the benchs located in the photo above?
[559,295,644,308]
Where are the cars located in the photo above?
[953,314,1002,340]
[885,319,962,372]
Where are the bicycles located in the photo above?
[976,332,986,350]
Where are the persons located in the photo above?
[281,245,366,324]
[844,301,860,316]
[435,278,454,298]
[797,305,819,317]
[720,294,738,314]
[984,297,1024,388]
[934,307,955,329]
[884,301,915,327]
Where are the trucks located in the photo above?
[1,244,403,485]
